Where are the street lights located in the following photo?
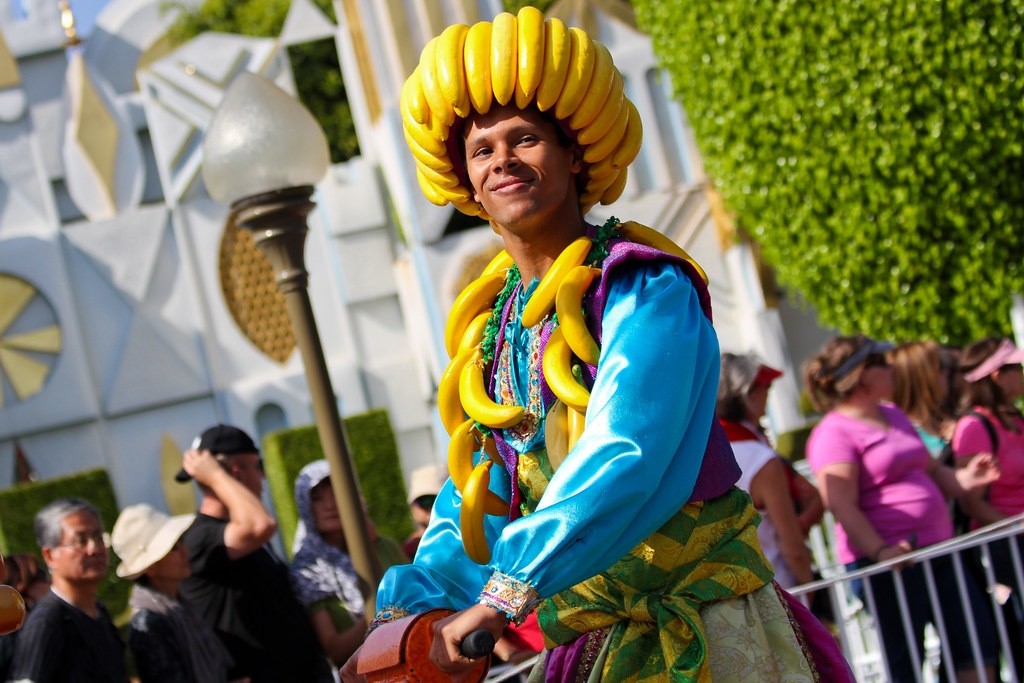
[202,67,376,624]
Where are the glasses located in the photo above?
[853,354,891,382]
[413,496,438,510]
[51,530,113,554]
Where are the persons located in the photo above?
[0,423,377,683]
[402,494,544,683]
[716,334,1024,683]
[339,7,857,683]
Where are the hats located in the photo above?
[175,423,255,482]
[820,339,896,387]
[111,503,199,577]
[408,462,450,503]
[962,339,1024,382]
[745,362,783,391]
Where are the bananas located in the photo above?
[617,220,708,282]
[437,236,601,565]
